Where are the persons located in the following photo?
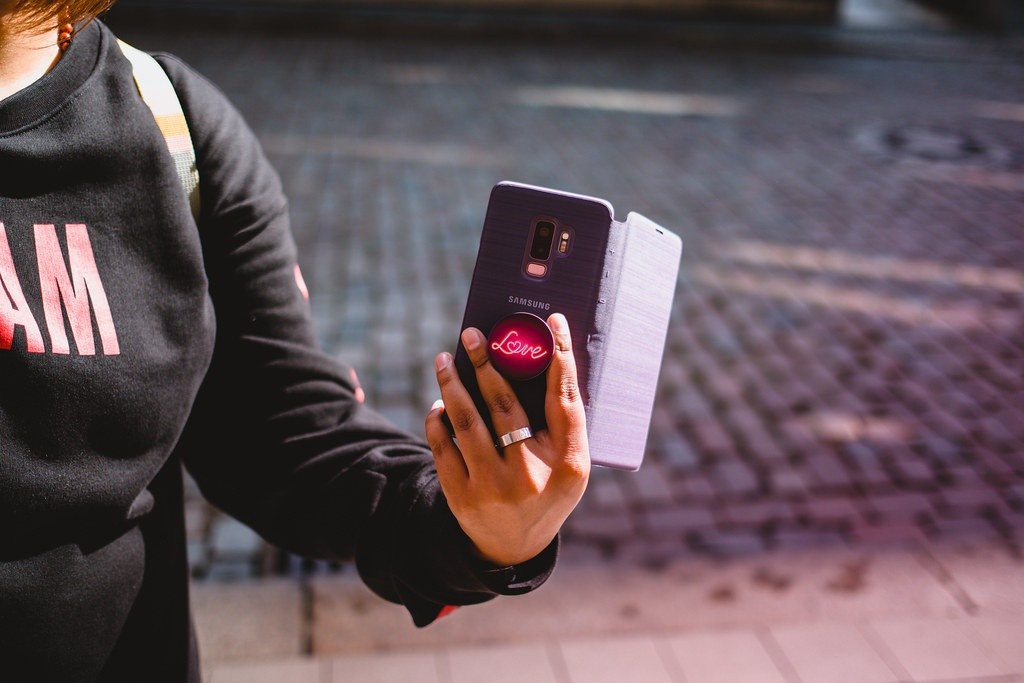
[0,1,591,683]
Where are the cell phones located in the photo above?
[436,181,682,471]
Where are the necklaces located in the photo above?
[55,3,74,55]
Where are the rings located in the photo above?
[500,428,531,447]
